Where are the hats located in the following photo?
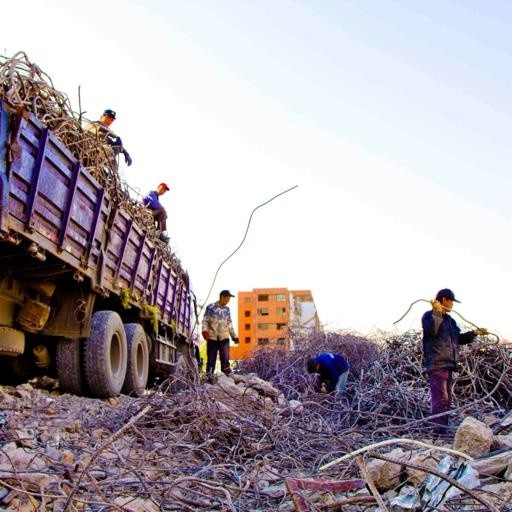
[436,288,462,304]
[220,289,235,298]
[103,109,116,119]
[160,182,170,191]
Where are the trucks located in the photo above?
[0,89,203,399]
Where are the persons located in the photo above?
[202,289,240,384]
[421,288,488,440]
[85,109,132,168]
[145,183,172,242]
[306,351,351,408]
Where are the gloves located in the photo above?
[430,299,443,313]
[124,152,132,166]
[116,136,122,145]
[476,326,488,334]
[202,331,210,341]
